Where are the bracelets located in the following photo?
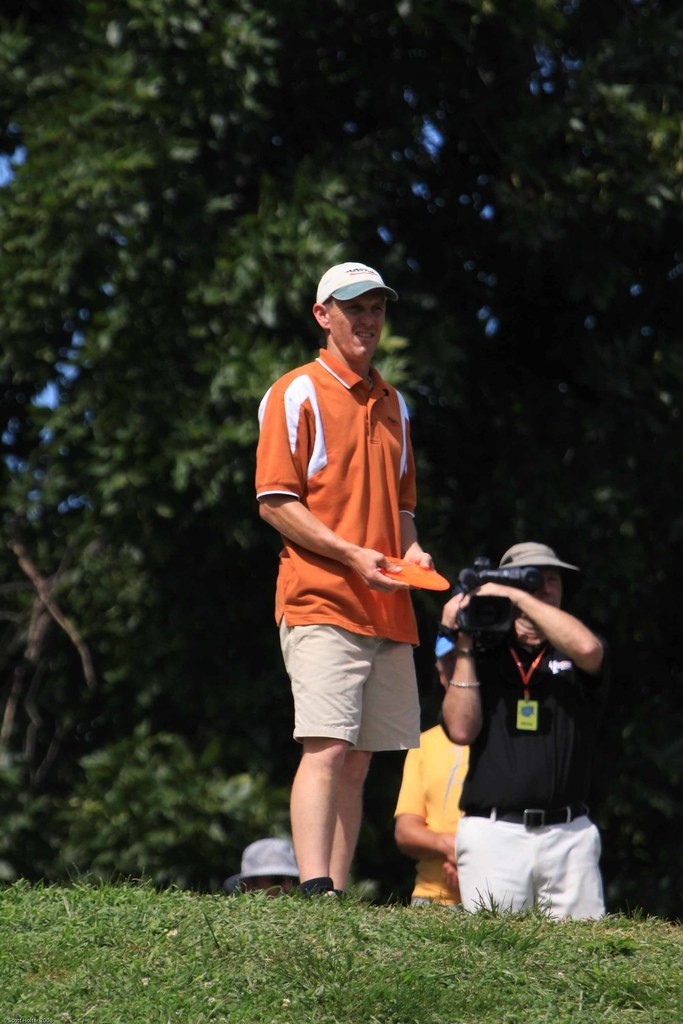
[447,679,482,689]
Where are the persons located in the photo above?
[392,637,470,913]
[254,261,452,910]
[221,836,300,901]
[433,543,607,921]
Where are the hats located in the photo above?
[316,262,398,308]
[226,838,302,890]
[432,634,454,657]
[499,543,578,583]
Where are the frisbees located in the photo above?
[379,555,450,590]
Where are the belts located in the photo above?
[465,801,585,827]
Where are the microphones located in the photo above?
[459,568,483,592]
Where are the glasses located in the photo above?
[256,876,298,888]
